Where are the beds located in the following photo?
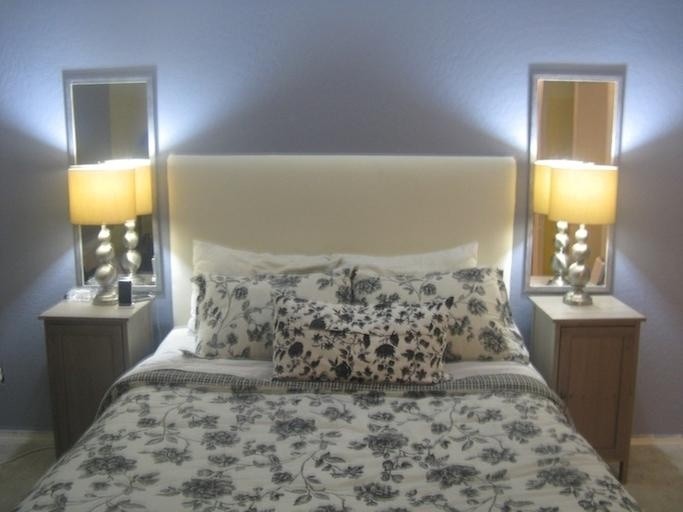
[15,155,640,512]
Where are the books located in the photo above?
[591,257,605,284]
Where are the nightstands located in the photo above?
[37,293,157,463]
[527,294,647,482]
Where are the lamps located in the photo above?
[105,158,153,286]
[63,161,136,306]
[533,160,587,287]
[549,162,620,307]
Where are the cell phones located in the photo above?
[119,281,131,305]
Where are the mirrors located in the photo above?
[64,73,164,293]
[522,72,623,294]
